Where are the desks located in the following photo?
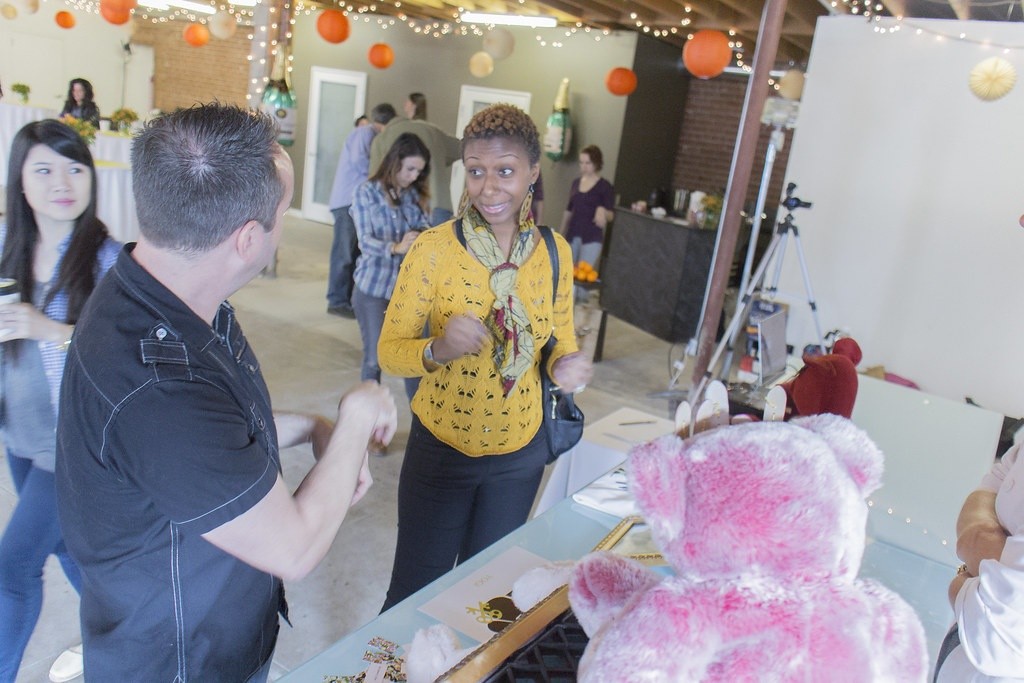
[591,205,776,365]
[263,434,965,683]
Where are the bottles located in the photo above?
[542,79,574,161]
[256,41,296,146]
[0,278,21,303]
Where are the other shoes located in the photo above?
[47,643,85,681]
[328,304,356,320]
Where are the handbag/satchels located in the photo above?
[538,222,584,464]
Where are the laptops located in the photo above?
[757,309,797,391]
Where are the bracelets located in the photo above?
[56,325,75,351]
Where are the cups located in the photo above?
[99,120,111,130]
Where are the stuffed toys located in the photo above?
[774,336,862,418]
[405,561,574,683]
[567,414,929,682]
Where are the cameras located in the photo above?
[782,196,811,210]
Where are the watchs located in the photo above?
[424,339,449,367]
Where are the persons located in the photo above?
[934,215,1023,683]
[55,101,399,683]
[529,170,544,225]
[560,146,615,336]
[369,104,464,227]
[326,116,380,318]
[1,119,121,683]
[351,133,434,456]
[376,103,592,614]
[405,93,427,119]
[59,78,100,129]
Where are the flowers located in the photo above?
[58,113,96,146]
[111,108,139,123]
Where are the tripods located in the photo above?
[689,214,829,410]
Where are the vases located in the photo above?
[117,121,130,134]
